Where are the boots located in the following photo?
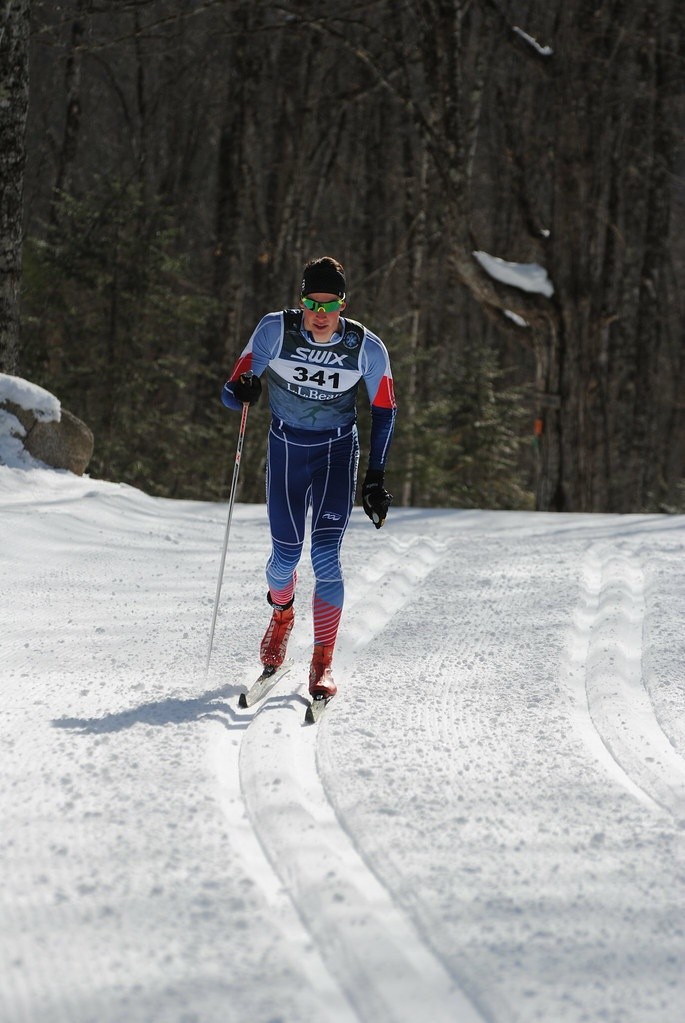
[309,642,337,695]
[260,591,295,666]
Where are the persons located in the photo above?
[220,257,398,701]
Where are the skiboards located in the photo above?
[238,665,328,722]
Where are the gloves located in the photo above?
[233,375,263,408]
[362,475,392,529]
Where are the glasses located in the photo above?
[301,298,342,313]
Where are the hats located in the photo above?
[302,256,346,299]
